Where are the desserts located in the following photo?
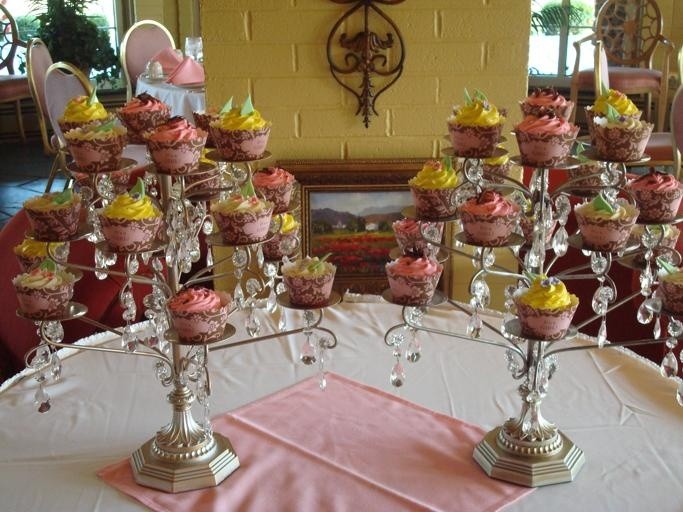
[12,83,332,315]
[386,82,683,310]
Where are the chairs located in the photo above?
[0,3,175,194]
[1,191,172,370]
[570,2,674,131]
[517,41,682,403]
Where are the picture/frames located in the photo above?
[300,183,452,297]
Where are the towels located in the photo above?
[88,369,541,510]
[164,55,204,85]
[146,46,183,75]
